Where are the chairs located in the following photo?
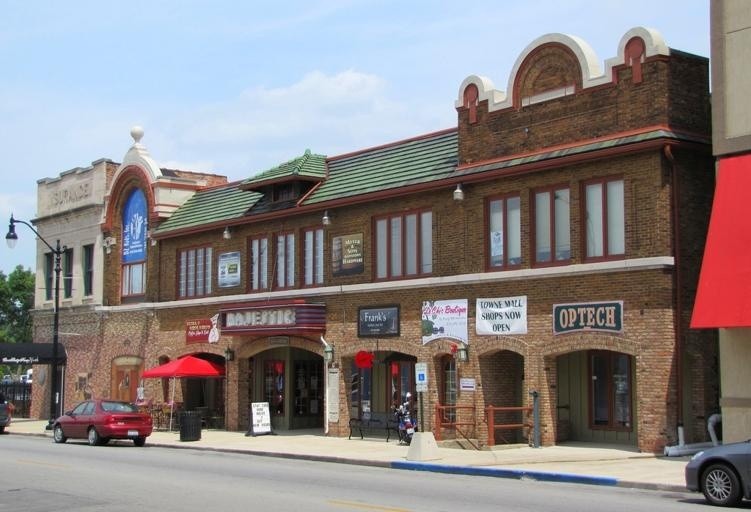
[196,404,225,431]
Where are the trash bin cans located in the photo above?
[179,411,203,440]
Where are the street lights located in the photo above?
[6,215,67,431]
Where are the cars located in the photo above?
[685,439,750,506]
[52,398,154,447]
[0,394,14,433]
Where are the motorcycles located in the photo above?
[390,392,419,446]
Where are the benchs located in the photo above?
[349,418,402,441]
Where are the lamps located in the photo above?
[454,182,466,201]
[225,346,235,361]
[223,225,232,239]
[322,209,332,226]
[457,348,469,363]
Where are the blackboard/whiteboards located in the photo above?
[252,402,270,433]
